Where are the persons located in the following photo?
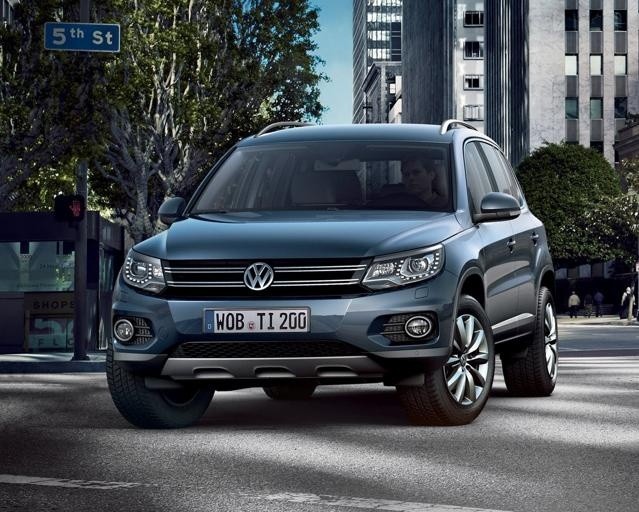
[594,290,604,317]
[568,291,581,318]
[400,155,448,208]
[621,287,636,319]
[583,292,593,318]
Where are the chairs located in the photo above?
[288,167,337,210]
[427,164,450,199]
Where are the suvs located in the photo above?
[105,116,558,425]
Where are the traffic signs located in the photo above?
[66,197,87,221]
[45,20,120,53]
